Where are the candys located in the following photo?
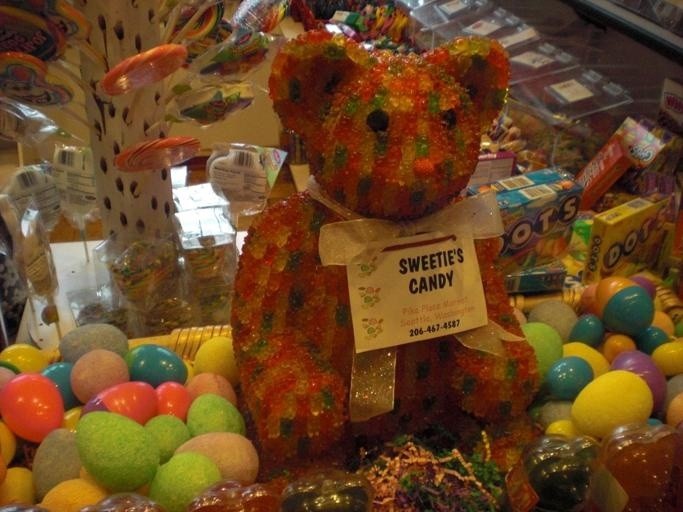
[0,0,683,512]
